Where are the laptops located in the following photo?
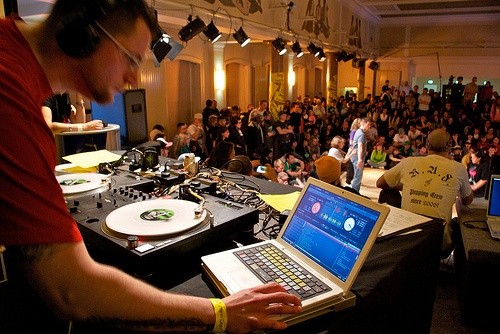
[199,177,390,321]
[485,175,500,238]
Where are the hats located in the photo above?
[314,155,340,184]
[220,107,231,116]
[278,111,285,115]
[426,128,452,153]
[194,114,202,119]
[209,115,218,119]
[263,111,270,116]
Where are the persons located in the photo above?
[149,125,173,156]
[376,129,474,263]
[0,0,302,334]
[41,90,104,135]
[173,76,500,197]
[310,156,371,200]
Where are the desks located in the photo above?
[56,124,121,165]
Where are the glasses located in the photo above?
[93,18,147,73]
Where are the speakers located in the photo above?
[90,89,148,149]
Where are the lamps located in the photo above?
[316,44,327,63]
[177,5,207,43]
[351,55,365,69]
[306,41,320,58]
[148,23,184,68]
[343,51,355,63]
[270,31,288,56]
[289,38,303,58]
[232,19,251,48]
[202,17,223,45]
[335,49,348,62]
[368,58,379,71]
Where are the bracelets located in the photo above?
[70,126,72,132]
[78,102,85,107]
[209,299,227,333]
[78,124,82,131]
